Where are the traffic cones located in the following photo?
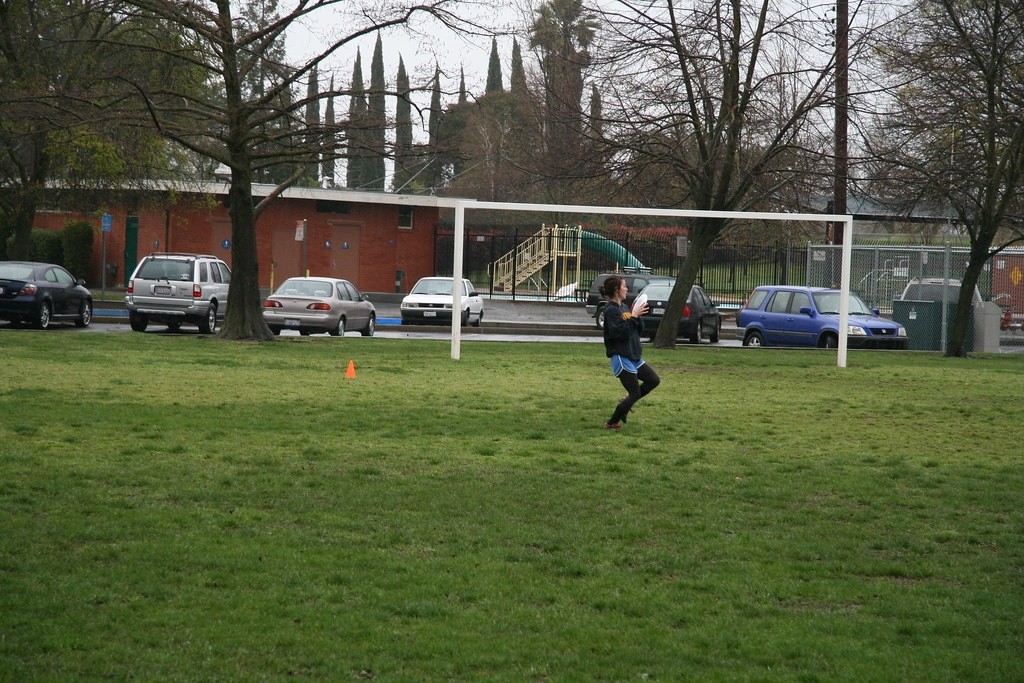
[345,361,356,380]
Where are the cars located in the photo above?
[262,276,377,337]
[1,261,94,330]
[630,283,723,344]
[400,276,485,327]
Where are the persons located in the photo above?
[597,274,661,428]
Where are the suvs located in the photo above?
[735,284,910,349]
[897,277,983,307]
[125,252,231,334]
[586,273,678,330]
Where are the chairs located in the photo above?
[286,289,297,294]
[313,290,327,297]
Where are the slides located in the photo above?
[578,231,650,274]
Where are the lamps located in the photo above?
[153,240,159,249]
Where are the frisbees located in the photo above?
[631,294,647,317]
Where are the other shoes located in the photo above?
[604,423,621,429]
[622,413,627,423]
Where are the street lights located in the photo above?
[902,123,955,353]
